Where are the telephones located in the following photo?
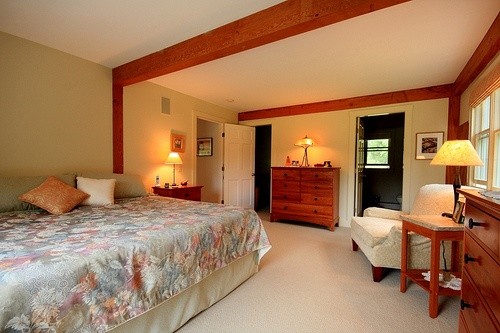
[155,176,160,187]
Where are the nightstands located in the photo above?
[152,184,204,202]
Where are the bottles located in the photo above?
[285,156,291,166]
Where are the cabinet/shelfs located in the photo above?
[456,186,500,333]
[270,166,340,233]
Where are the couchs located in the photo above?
[349,183,482,283]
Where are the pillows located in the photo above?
[74,176,116,207]
[17,177,91,216]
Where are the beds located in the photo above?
[0,194,272,333]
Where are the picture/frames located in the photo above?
[452,201,465,223]
[197,137,213,157]
[414,131,444,160]
[170,133,186,154]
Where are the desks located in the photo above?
[399,213,463,319]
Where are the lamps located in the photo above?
[294,135,315,168]
[430,140,485,223]
[165,152,183,186]
